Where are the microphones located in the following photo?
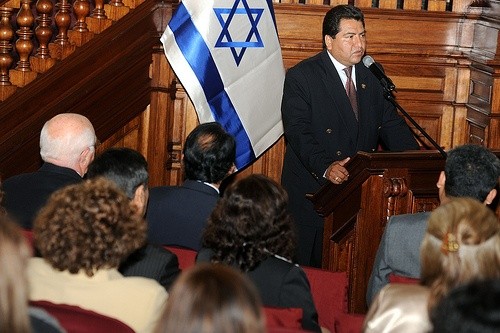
[361,56,396,90]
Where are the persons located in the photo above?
[87,147,182,292]
[25,178,168,333]
[0,203,34,333]
[281,4,420,268]
[194,174,332,333]
[366,144,500,308]
[0,113,97,230]
[363,196,500,333]
[144,122,236,251]
[158,261,266,333]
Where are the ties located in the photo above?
[343,66,358,121]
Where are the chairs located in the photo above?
[265,266,367,333]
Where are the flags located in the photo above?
[160,0,285,172]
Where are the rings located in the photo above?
[335,176,339,180]
